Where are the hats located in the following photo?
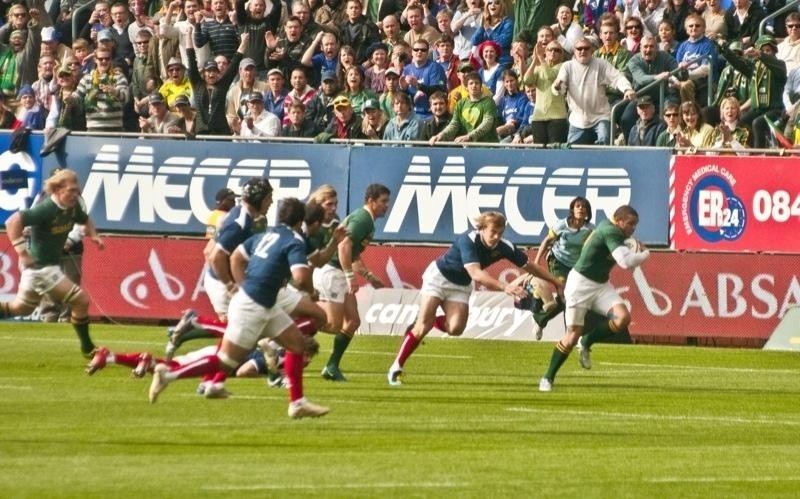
[455,60,475,73]
[40,25,55,41]
[239,57,258,69]
[97,30,115,43]
[321,71,338,84]
[248,92,264,101]
[754,34,779,54]
[333,95,351,108]
[149,92,164,104]
[637,96,655,107]
[58,67,73,76]
[174,94,190,106]
[366,41,389,57]
[216,188,241,202]
[167,56,188,71]
[18,85,35,96]
[203,60,219,70]
[242,178,273,198]
[267,69,285,78]
[384,68,401,77]
[479,41,503,58]
[729,41,744,54]
[363,99,380,111]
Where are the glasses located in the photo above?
[547,47,563,54]
[58,72,73,78]
[625,25,643,30]
[110,11,127,17]
[168,68,182,72]
[663,112,680,118]
[13,12,27,19]
[687,23,702,28]
[66,60,81,68]
[413,47,429,54]
[575,46,591,51]
[137,41,151,44]
[95,56,112,63]
[486,0,502,7]
[335,99,348,104]
[785,23,800,29]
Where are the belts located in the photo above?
[1,91,19,101]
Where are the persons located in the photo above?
[80,2,110,38]
[414,91,453,146]
[548,4,586,60]
[144,16,181,91]
[379,67,400,118]
[675,101,714,156]
[137,93,178,139]
[336,45,357,83]
[657,105,680,146]
[304,70,338,130]
[185,26,249,141]
[620,17,650,56]
[352,98,389,146]
[231,91,281,143]
[236,0,281,81]
[601,13,621,31]
[45,55,80,128]
[399,0,437,31]
[755,0,786,35]
[55,0,92,32]
[402,5,442,49]
[158,57,191,112]
[63,47,129,131]
[7,4,27,29]
[701,97,752,156]
[428,72,498,146]
[450,0,482,61]
[616,0,627,18]
[363,42,400,93]
[41,26,72,62]
[150,198,331,418]
[707,33,787,148]
[313,96,362,144]
[160,0,209,69]
[166,203,351,361]
[301,31,340,69]
[32,55,56,110]
[282,68,317,129]
[540,205,651,393]
[0,168,104,359]
[190,56,226,109]
[433,0,460,14]
[584,0,616,42]
[382,92,429,147]
[448,61,494,116]
[471,0,514,68]
[338,0,381,62]
[312,0,349,31]
[726,0,765,48]
[656,20,680,58]
[312,184,390,380]
[623,0,670,36]
[524,196,600,340]
[495,69,529,143]
[676,14,719,81]
[701,41,750,125]
[199,177,272,400]
[88,336,319,377]
[591,23,632,105]
[513,0,575,41]
[380,15,406,59]
[436,9,452,34]
[91,2,136,67]
[434,33,461,92]
[291,0,323,37]
[627,95,667,146]
[510,38,534,91]
[226,58,270,133]
[509,83,534,144]
[195,0,238,63]
[400,39,446,121]
[310,187,340,246]
[771,66,800,148]
[166,95,197,140]
[551,37,635,145]
[14,86,49,131]
[477,41,507,105]
[71,38,89,60]
[341,66,377,118]
[25,169,86,323]
[264,16,311,90]
[524,41,569,144]
[281,101,319,138]
[388,211,565,388]
[776,12,800,75]
[610,36,689,139]
[130,30,152,112]
[361,0,396,23]
[81,30,129,77]
[50,65,87,131]
[204,189,241,238]
[0,9,41,98]
[663,0,699,43]
[128,0,153,56]
[537,25,554,50]
[701,0,728,40]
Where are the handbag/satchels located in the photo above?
[9,126,30,152]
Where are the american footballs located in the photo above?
[624,238,641,254]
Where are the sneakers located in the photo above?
[92,347,109,372]
[149,364,168,404]
[80,348,97,361]
[577,336,592,370]
[414,314,422,320]
[288,404,329,418]
[204,385,228,398]
[268,377,291,390]
[174,311,195,334]
[169,330,175,337]
[388,365,403,386]
[539,376,551,393]
[44,126,70,150]
[538,328,544,340]
[321,367,346,381]
[41,143,59,157]
[166,343,177,362]
[134,352,151,377]
